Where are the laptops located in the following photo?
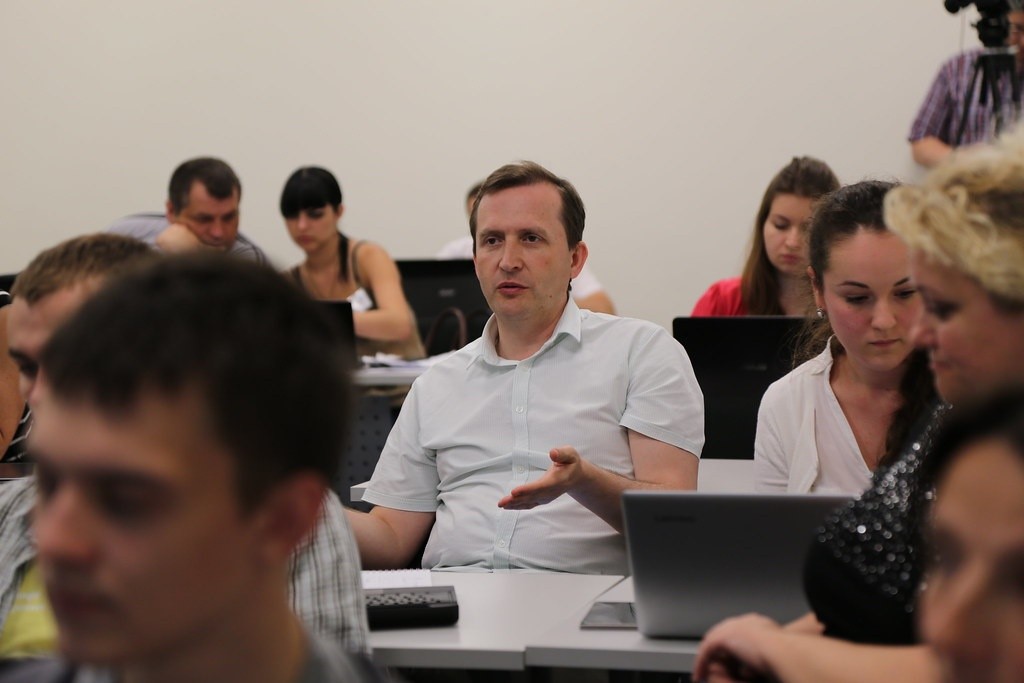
[666,316,811,430]
[618,490,861,640]
[315,298,366,372]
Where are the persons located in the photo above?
[281,168,423,360]
[342,162,705,573]
[691,157,840,318]
[754,179,931,497]
[0,235,387,683]
[919,380,1024,682]
[104,157,269,265]
[693,126,1024,683]
[909,0,1024,169]
[445,183,614,314]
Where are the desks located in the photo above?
[324,355,784,683]
